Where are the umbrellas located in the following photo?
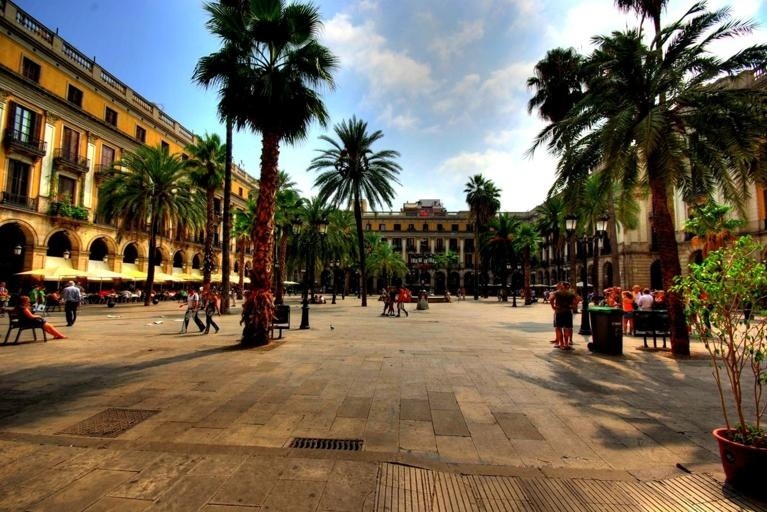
[16,264,250,291]
[284,282,300,284]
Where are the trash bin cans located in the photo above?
[417,290,428,310]
[587,307,625,355]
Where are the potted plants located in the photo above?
[666,233,766,504]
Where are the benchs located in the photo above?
[631,309,671,348]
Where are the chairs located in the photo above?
[267,305,290,339]
[0,305,47,346]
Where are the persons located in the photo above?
[314,295,324,304]
[284,285,297,296]
[0,280,252,339]
[381,286,465,317]
[519,281,713,350]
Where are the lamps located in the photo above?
[13,245,22,256]
[63,248,70,261]
